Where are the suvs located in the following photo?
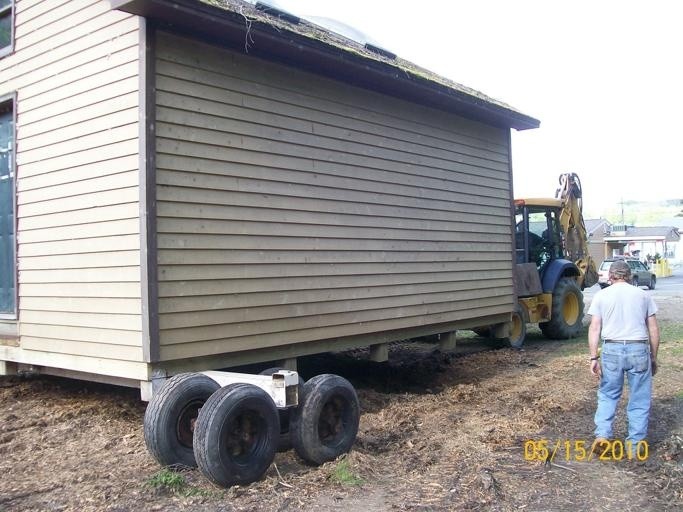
[600,255,656,292]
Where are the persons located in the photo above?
[588,259,658,461]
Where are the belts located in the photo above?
[604,339,645,345]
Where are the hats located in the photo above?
[609,260,630,276]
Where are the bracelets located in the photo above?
[590,355,599,360]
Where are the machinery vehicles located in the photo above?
[474,171,600,350]
